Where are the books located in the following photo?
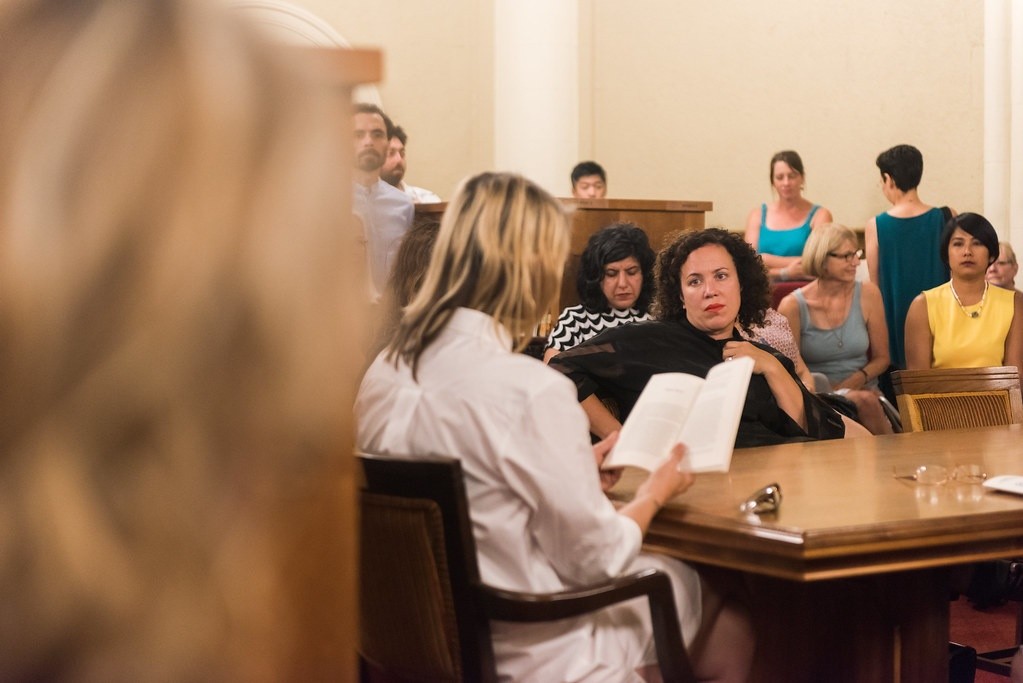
[599,354,756,476]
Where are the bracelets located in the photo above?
[779,266,789,282]
[860,368,869,385]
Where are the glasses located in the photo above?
[825,248,863,261]
[892,461,988,485]
[746,482,782,516]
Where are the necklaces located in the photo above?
[949,276,988,318]
[815,280,845,348]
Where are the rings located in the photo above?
[729,355,733,361]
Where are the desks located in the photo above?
[603,424,1023,683]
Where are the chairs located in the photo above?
[352,451,701,683]
[892,364,1022,432]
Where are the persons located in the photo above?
[542,219,658,366]
[984,242,1020,290]
[355,218,443,394]
[376,126,407,192]
[863,143,959,408]
[904,212,1023,396]
[349,172,758,683]
[545,227,845,447]
[349,103,417,306]
[739,151,833,282]
[776,222,893,435]
[572,159,607,200]
